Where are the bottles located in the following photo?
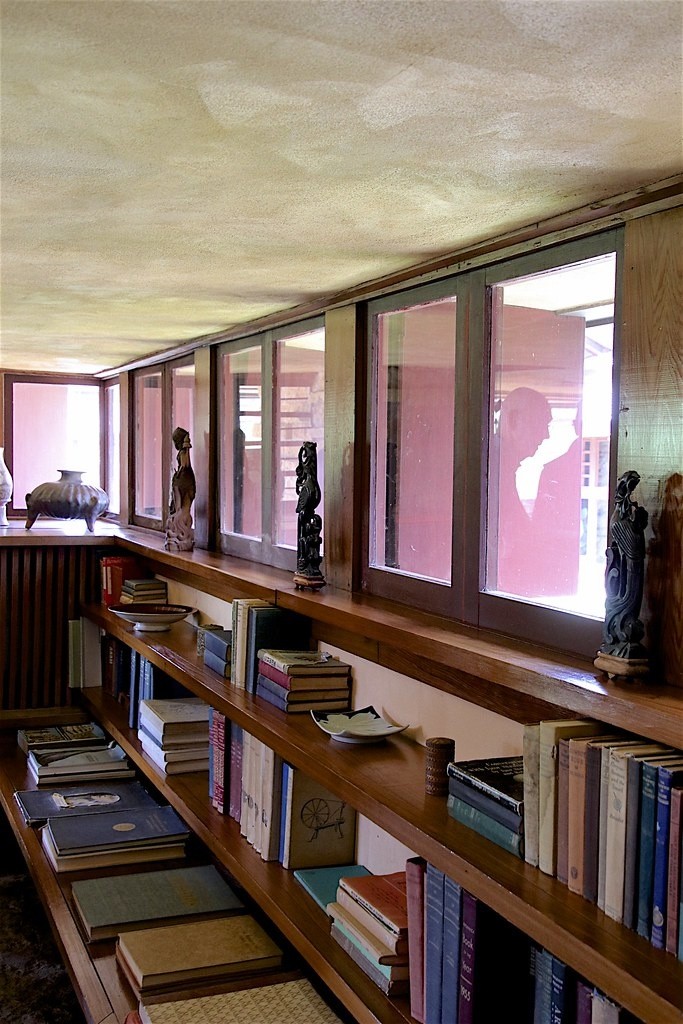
[0,447,13,506]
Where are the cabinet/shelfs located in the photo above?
[1,517,683,1024]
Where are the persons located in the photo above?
[487,386,581,596]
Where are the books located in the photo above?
[14,721,340,1024]
[68,553,355,870]
[324,719,683,1024]
[293,864,373,918]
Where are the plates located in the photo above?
[310,705,410,744]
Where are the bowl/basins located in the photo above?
[107,603,198,631]
